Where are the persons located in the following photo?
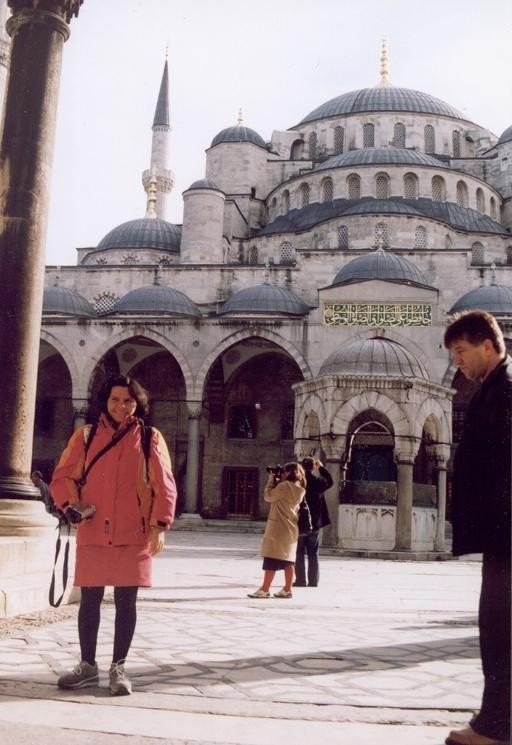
[292,455,334,588]
[247,462,307,598]
[51,373,180,697]
[438,307,511,745]
[29,470,53,509]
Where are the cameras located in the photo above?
[65,502,97,525]
[264,463,283,479]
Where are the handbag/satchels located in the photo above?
[53,485,83,527]
[297,501,315,538]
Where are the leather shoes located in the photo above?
[444,727,510,745]
[293,583,317,587]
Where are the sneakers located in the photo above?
[57,659,100,689]
[247,589,271,599]
[108,663,133,696]
[273,588,292,598]
